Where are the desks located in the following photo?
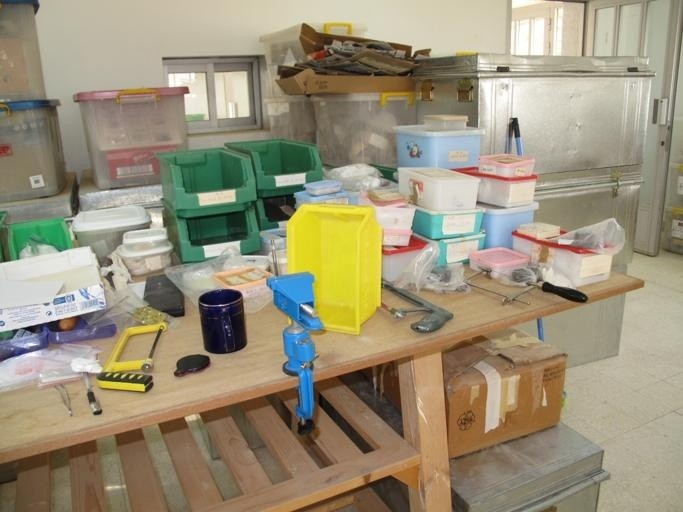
[2,253,648,512]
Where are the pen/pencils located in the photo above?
[83,372,103,415]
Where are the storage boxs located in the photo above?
[258,22,654,461]
[0,2,190,275]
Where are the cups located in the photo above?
[199,289,247,353]
